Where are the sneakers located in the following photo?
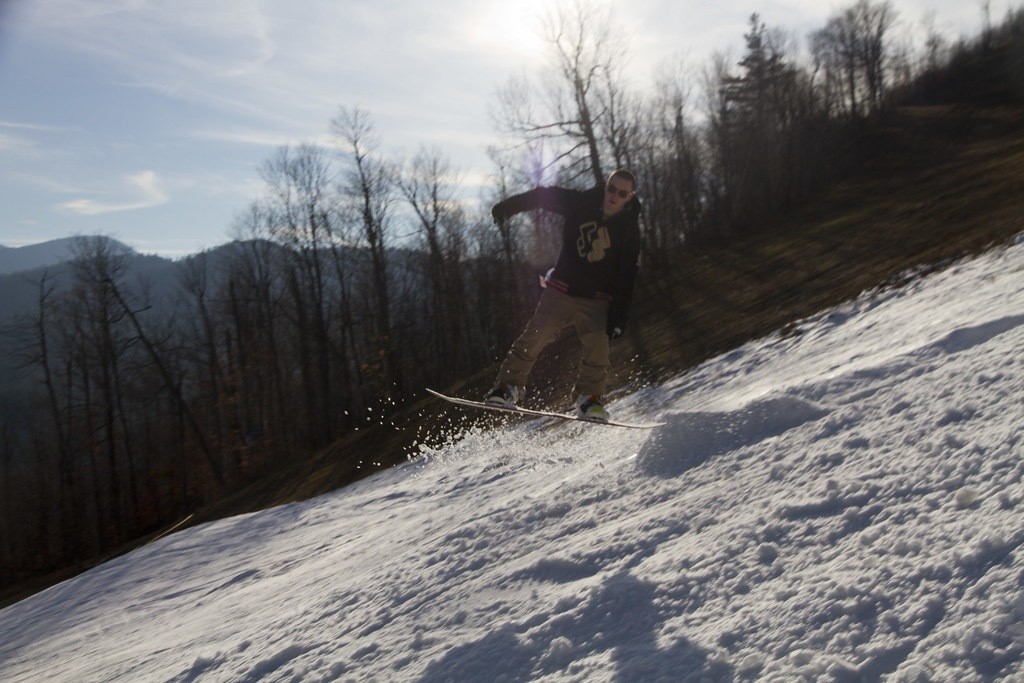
[485,386,518,407]
[575,401,611,421]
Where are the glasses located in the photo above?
[608,183,632,198]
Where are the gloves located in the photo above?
[492,204,508,223]
[607,313,627,338]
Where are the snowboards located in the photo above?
[424,386,668,429]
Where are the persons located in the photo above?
[483,166,640,420]
